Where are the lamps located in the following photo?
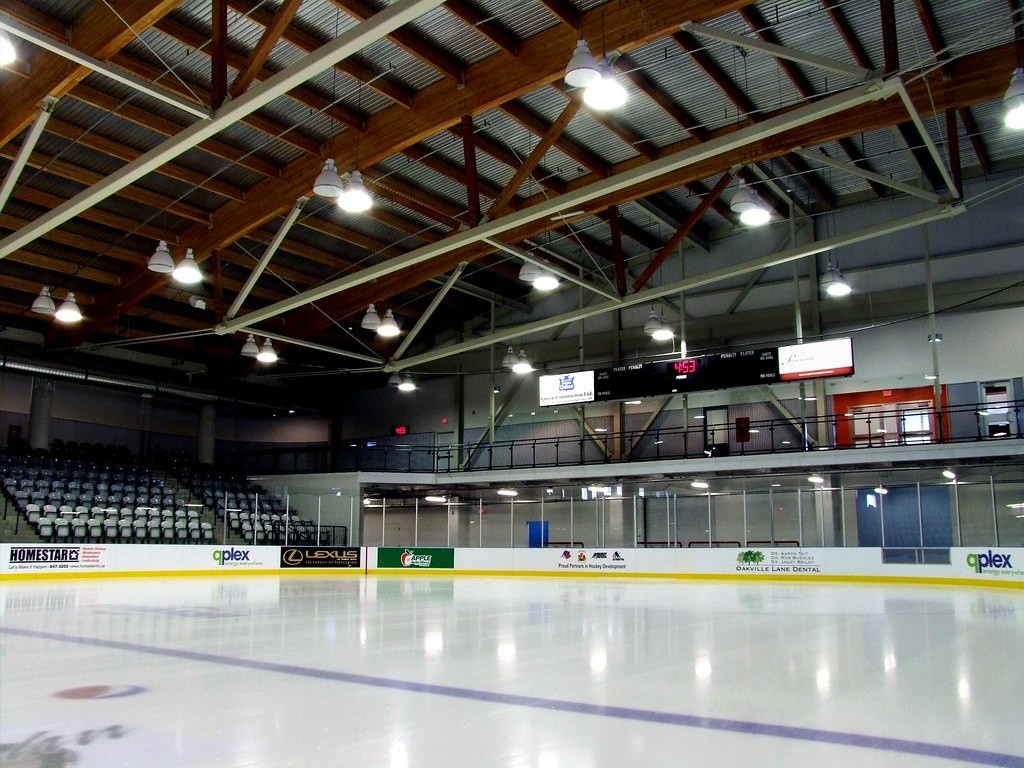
[943,468,956,479]
[397,368,416,390]
[875,484,888,494]
[821,163,841,287]
[651,226,675,340]
[808,474,824,483]
[195,298,205,309]
[1001,67,1024,108]
[389,374,401,387]
[256,337,277,362]
[927,333,942,342]
[692,479,708,488]
[512,311,533,374]
[564,0,598,86]
[501,311,518,367]
[424,496,446,503]
[147,211,174,274]
[588,483,607,493]
[497,487,518,496]
[361,223,380,329]
[337,50,373,212]
[189,285,200,307]
[547,487,553,495]
[313,6,344,198]
[54,275,83,321]
[583,0,627,109]
[241,334,259,357]
[378,200,401,338]
[519,132,542,283]
[643,216,660,334]
[172,219,203,284]
[826,165,851,298]
[532,155,559,290]
[31,270,56,315]
[729,44,756,212]
[739,56,771,225]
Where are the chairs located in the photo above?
[0,439,329,545]
